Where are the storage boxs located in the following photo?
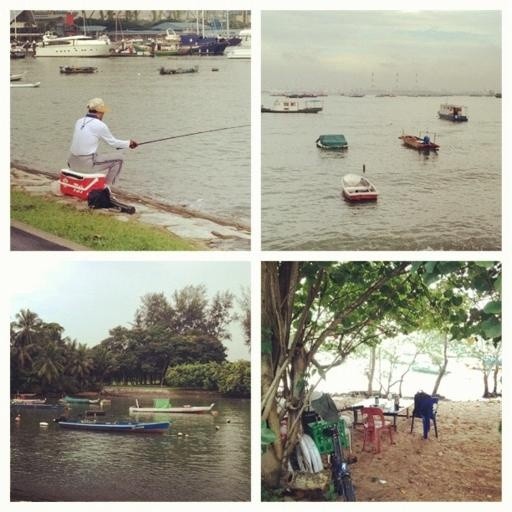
[60,169,106,200]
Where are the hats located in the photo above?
[86,98,107,113]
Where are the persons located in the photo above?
[15,414,22,421]
[423,135,431,144]
[67,97,139,202]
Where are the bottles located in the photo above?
[394,395,399,411]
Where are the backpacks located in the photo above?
[414,390,433,438]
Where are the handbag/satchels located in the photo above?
[88,188,113,208]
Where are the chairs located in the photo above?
[410,397,439,438]
[361,407,393,453]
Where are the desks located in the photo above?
[351,398,415,432]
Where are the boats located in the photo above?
[160,66,196,75]
[317,132,347,149]
[10,72,41,88]
[12,398,53,408]
[340,172,378,201]
[12,9,250,60]
[400,132,438,150]
[436,104,467,121]
[53,415,169,432]
[129,402,214,415]
[59,65,97,74]
[64,396,111,415]
[262,97,322,113]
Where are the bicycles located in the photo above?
[326,426,356,502]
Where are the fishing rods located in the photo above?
[116,124,251,151]
